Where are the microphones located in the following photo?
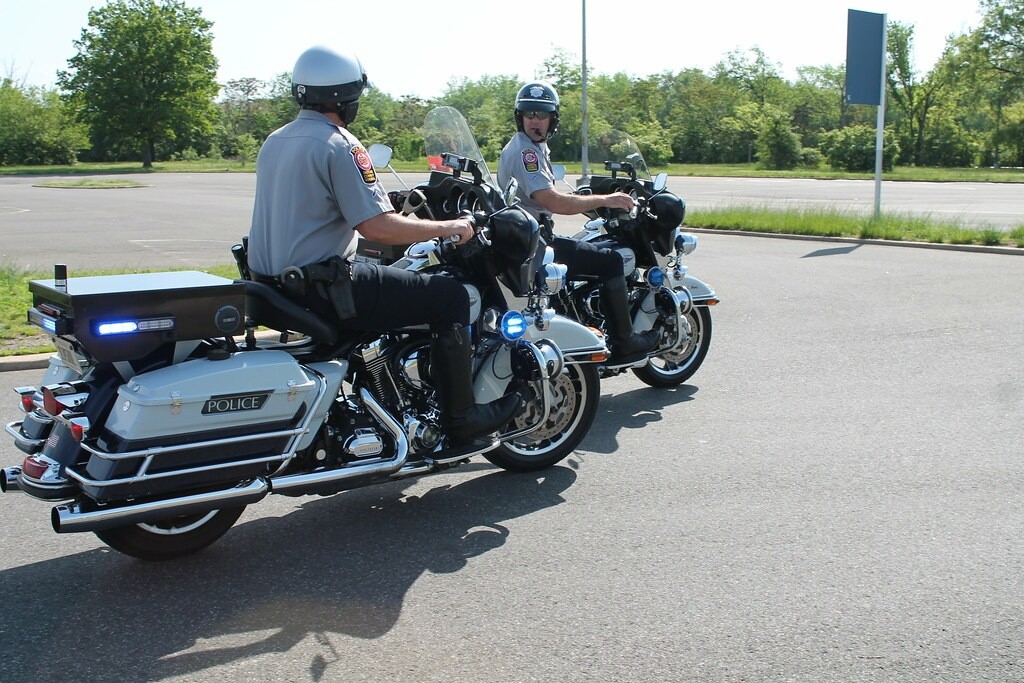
[535,128,557,140]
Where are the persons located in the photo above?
[497,81,661,360]
[246,45,526,448]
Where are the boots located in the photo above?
[598,275,661,365]
[430,325,523,447]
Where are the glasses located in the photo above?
[522,111,550,120]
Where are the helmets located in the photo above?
[514,80,560,143]
[291,44,367,125]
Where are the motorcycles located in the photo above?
[351,126,720,390]
[0,105,609,562]
